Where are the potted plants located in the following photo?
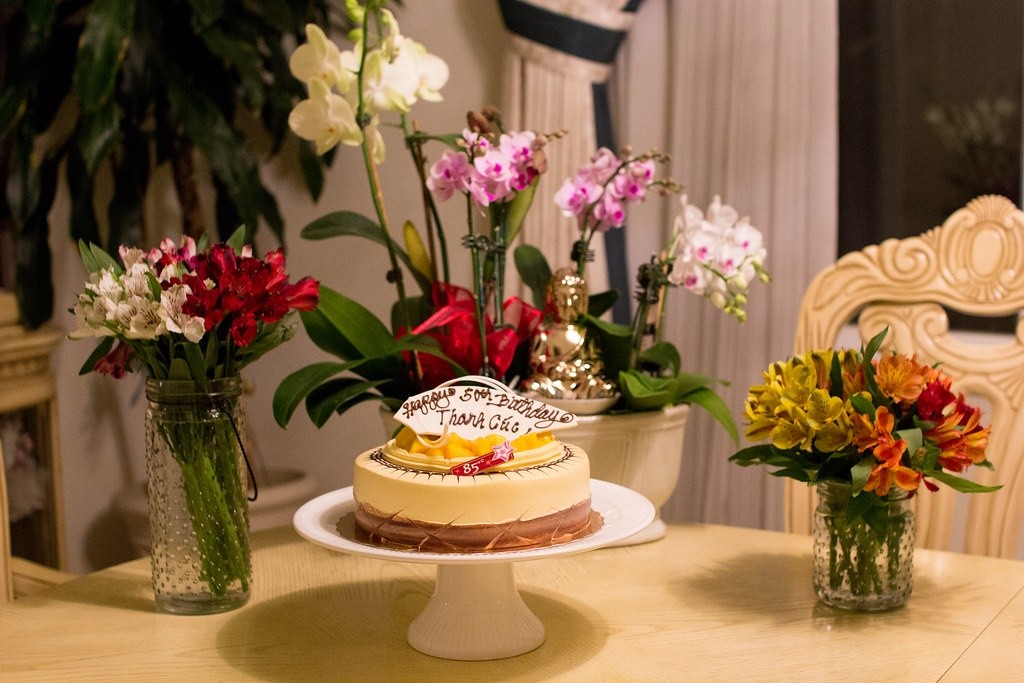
[1,0,415,556]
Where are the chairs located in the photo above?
[785,196,1024,562]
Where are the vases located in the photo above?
[548,402,690,548]
[812,482,913,611]
[142,375,250,615]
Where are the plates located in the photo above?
[520,392,621,413]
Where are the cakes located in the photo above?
[352,376,592,552]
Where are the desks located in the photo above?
[0,524,1024,683]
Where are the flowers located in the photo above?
[727,325,1004,596]
[65,221,321,596]
[272,0,771,455]
